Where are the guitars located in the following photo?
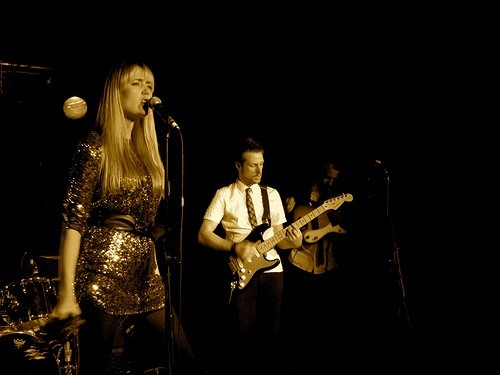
[228,193,353,290]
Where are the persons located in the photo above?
[287,153,349,375]
[150,180,176,264]
[197,137,303,375]
[48,58,196,375]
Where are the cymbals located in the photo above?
[40,256,59,259]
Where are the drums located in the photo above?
[0,277,58,331]
[0,331,60,375]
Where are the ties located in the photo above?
[310,217,325,267]
[245,188,258,229]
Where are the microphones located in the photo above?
[148,96,180,131]
[374,159,388,175]
